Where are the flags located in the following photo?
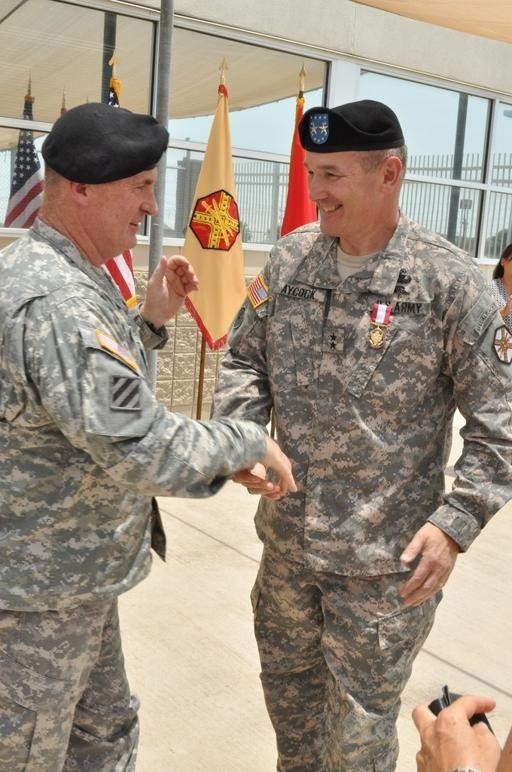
[281,96,317,236]
[101,79,137,313]
[4,101,47,227]
[181,86,248,351]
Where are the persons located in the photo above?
[412,693,511,772]
[1,103,300,772]
[492,244,512,331]
[215,99,512,772]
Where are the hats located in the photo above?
[298,100,404,152]
[41,102,169,184]
